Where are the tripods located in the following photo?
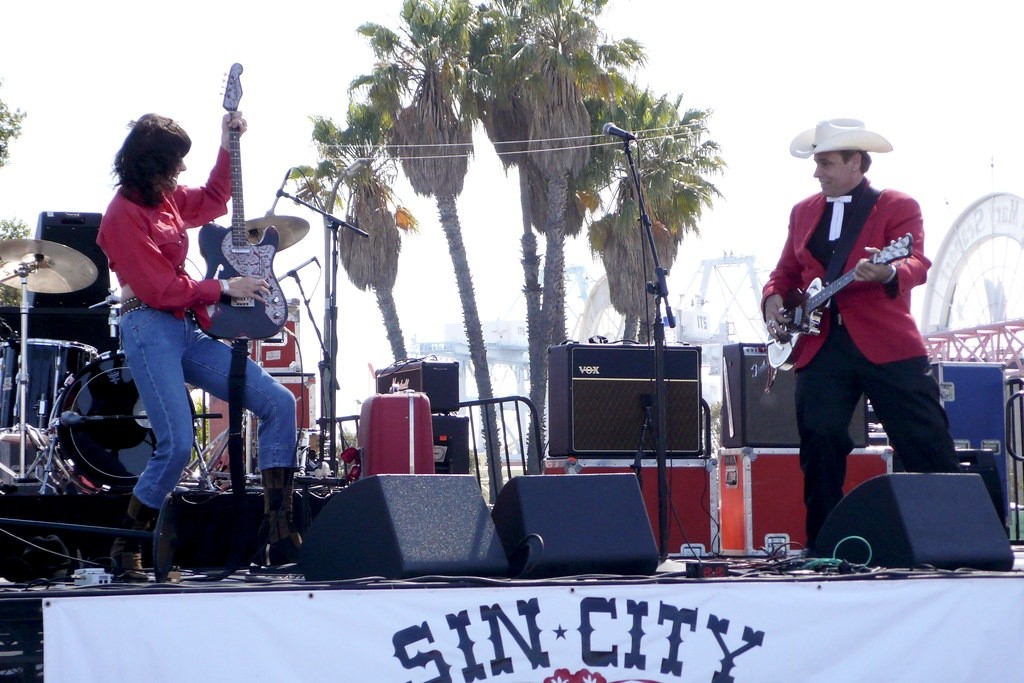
[0,267,71,480]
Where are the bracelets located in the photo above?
[220,279,229,294]
[881,264,896,284]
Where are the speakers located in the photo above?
[721,342,869,448]
[375,361,460,415]
[431,416,470,473]
[548,343,703,458]
[0,211,119,354]
[954,448,1006,531]
[490,473,659,578]
[812,472,1015,571]
[296,473,511,582]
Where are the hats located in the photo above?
[788,116,896,157]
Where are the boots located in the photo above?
[108,494,155,587]
[252,465,310,552]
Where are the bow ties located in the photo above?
[824,196,853,242]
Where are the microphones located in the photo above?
[265,168,291,217]
[603,122,636,140]
[59,411,82,426]
[275,258,315,282]
[642,392,654,431]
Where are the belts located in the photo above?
[118,296,152,315]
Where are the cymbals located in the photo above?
[0,238,99,294]
[244,214,311,252]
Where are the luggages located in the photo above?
[360,384,436,474]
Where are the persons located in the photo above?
[761,119,954,558]
[97,112,304,582]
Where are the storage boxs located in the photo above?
[931,361,1012,527]
[542,460,722,557]
[210,299,316,476]
[717,447,893,557]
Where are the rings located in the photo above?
[771,321,777,325]
[244,123,247,126]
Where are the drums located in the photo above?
[0,338,196,495]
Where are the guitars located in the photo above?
[766,232,914,372]
[194,62,289,341]
